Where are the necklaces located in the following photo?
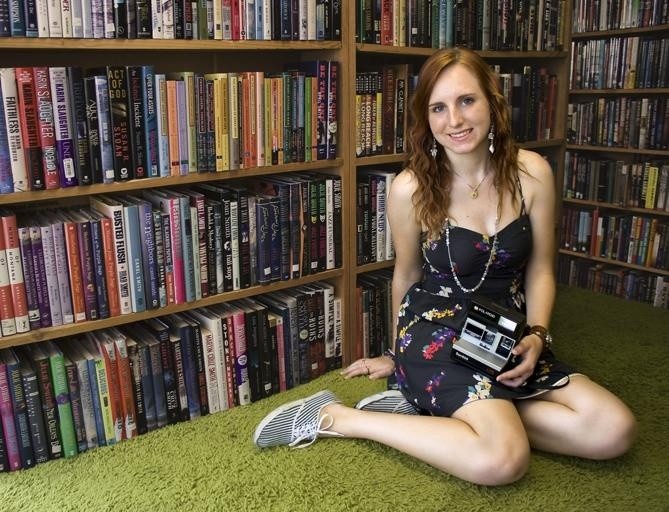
[442,158,501,294]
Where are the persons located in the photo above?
[254,48,637,487]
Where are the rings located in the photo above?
[360,359,371,375]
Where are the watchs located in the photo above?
[528,325,553,352]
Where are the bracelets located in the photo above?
[384,348,396,361]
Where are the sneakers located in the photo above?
[354,390,420,414]
[254,389,336,447]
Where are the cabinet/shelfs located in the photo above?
[0,3,352,368]
[558,3,668,274]
[350,4,567,365]
